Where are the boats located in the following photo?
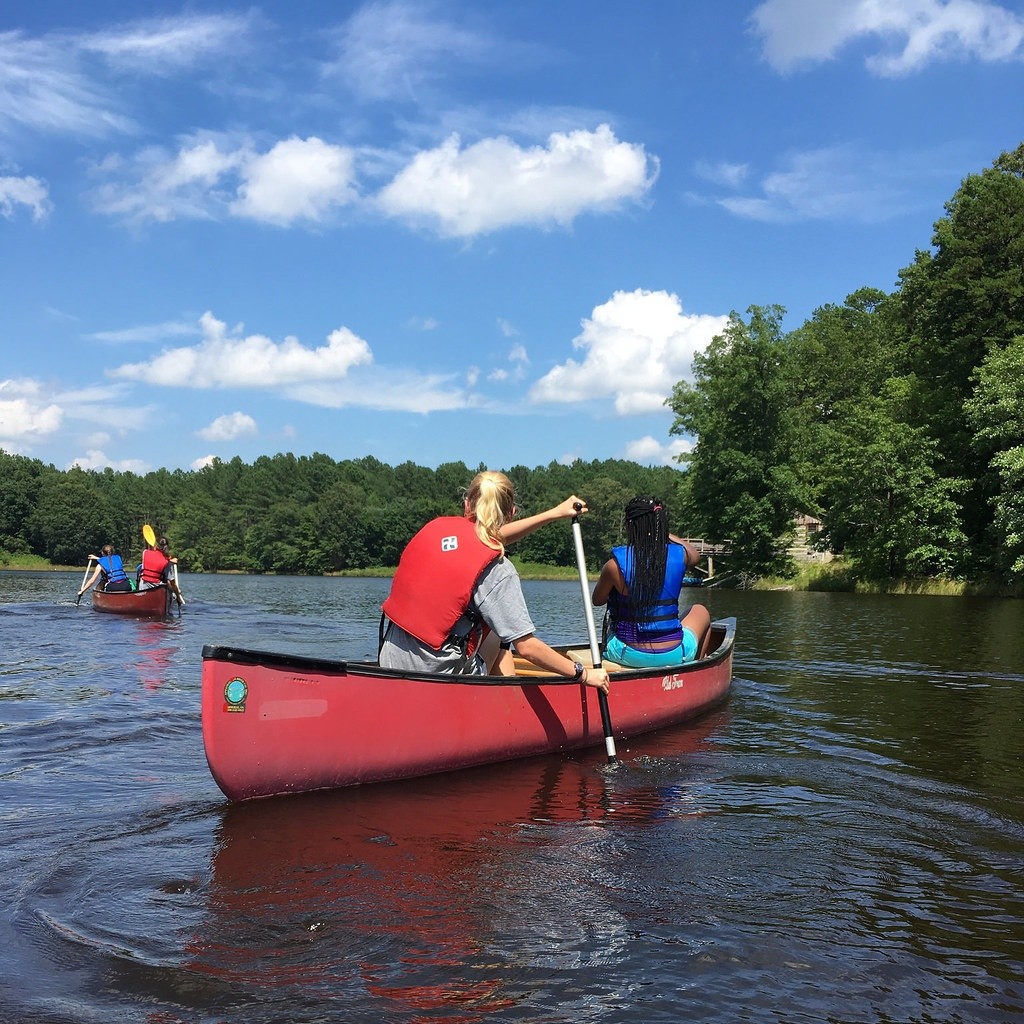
[92,580,172,616]
[201,604,737,803]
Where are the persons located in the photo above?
[592,493,713,667]
[380,470,610,696]
[136,536,182,595]
[77,540,131,597]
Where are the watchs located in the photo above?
[574,663,583,683]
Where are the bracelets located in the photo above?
[582,667,587,684]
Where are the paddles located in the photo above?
[75,555,93,605]
[142,524,187,606]
[570,501,621,764]
